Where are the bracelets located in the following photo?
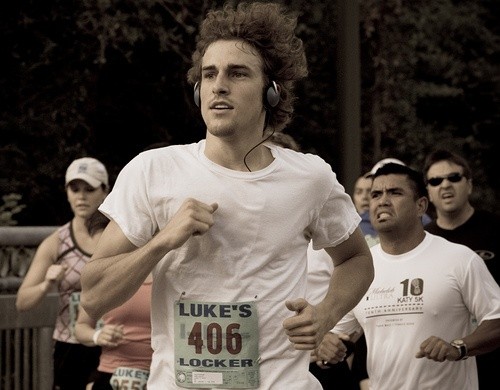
[92,330,101,344]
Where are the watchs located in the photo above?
[452,340,469,361]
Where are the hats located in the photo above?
[65,158,108,188]
[365,157,406,178]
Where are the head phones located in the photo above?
[193,78,279,109]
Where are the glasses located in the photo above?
[426,174,465,186]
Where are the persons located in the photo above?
[13,133,500,390]
[79,1,375,390]
